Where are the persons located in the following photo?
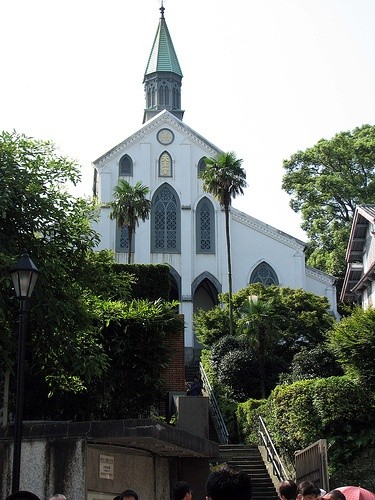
[189,374,204,396]
[279,480,298,500]
[296,481,326,500]
[172,481,193,500]
[327,490,346,500]
[206,463,252,500]
[50,494,66,500]
[113,490,138,500]
[6,491,40,500]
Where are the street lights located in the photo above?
[12,246,40,494]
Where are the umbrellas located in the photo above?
[323,486,375,500]
